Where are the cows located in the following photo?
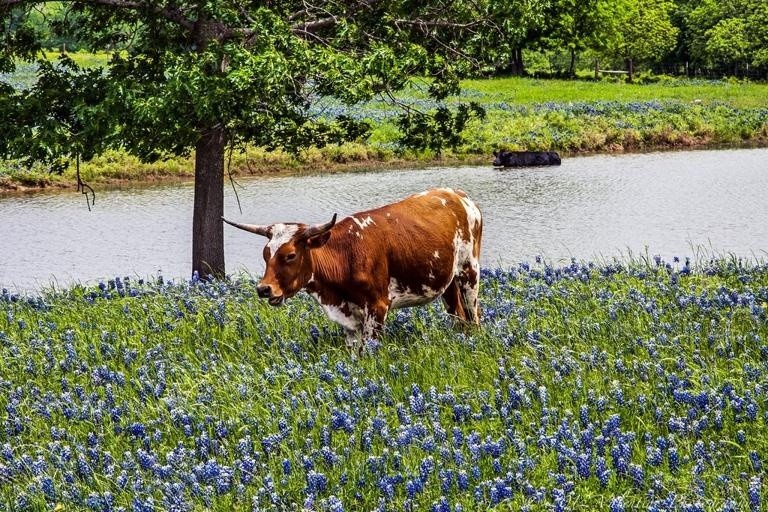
[492,148,550,168]
[548,152,562,166]
[219,185,483,355]
